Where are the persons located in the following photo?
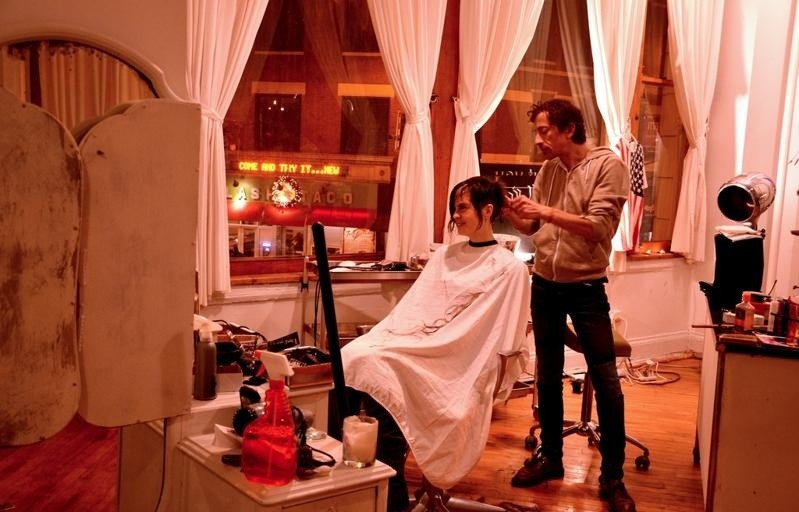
[329,175,531,511]
[495,98,635,511]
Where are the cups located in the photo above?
[343,415,379,468]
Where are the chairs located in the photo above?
[357,320,533,512]
[525,297,650,469]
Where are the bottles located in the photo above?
[767,293,779,332]
[736,292,755,333]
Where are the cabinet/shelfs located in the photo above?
[122,356,335,511]
[177,432,399,512]
[694,286,799,512]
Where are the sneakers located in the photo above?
[598,475,635,512]
[511,452,564,487]
